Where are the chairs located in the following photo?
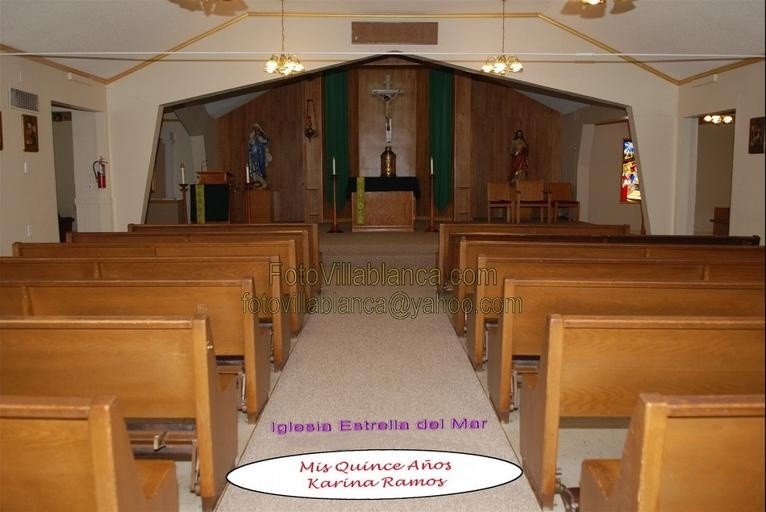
[486,181,581,225]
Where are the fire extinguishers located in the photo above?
[93,157,109,189]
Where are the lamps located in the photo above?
[479,0,524,77]
[263,0,304,76]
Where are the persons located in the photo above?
[509,129,530,186]
[373,90,402,131]
[248,122,273,190]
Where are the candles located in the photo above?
[332,157,336,175]
[181,164,185,184]
[246,163,249,183]
[430,157,433,175]
